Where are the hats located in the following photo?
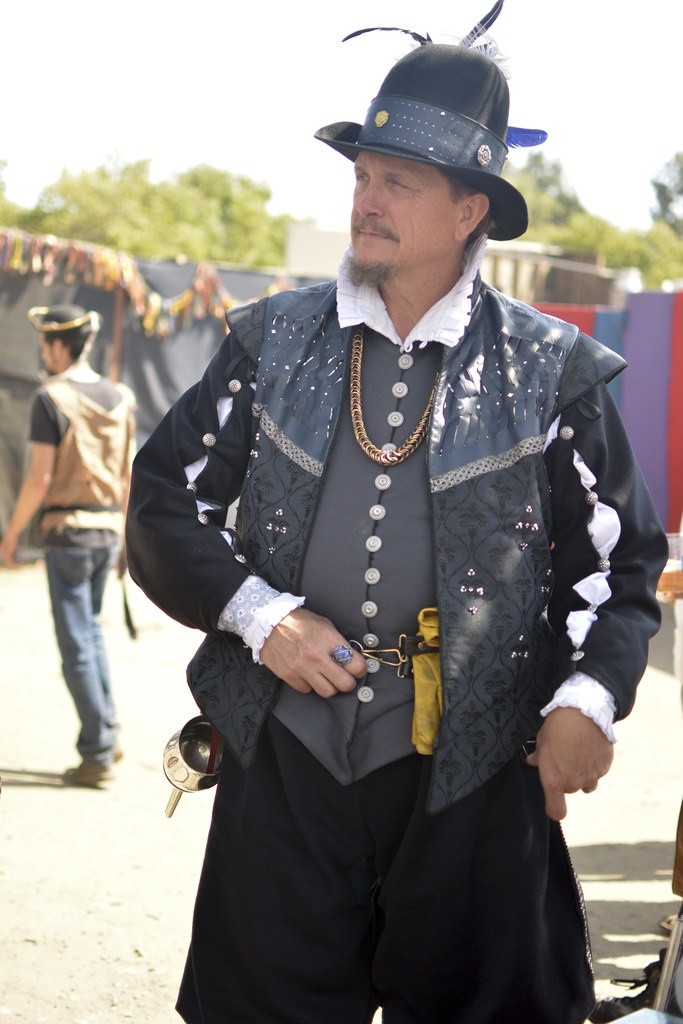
[27,304,100,332]
[314,0,548,241]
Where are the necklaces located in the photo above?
[350,324,440,466]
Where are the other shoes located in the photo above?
[61,759,115,790]
[112,744,123,763]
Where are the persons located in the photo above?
[125,45,670,1024]
[0,305,139,783]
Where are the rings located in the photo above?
[331,644,354,667]
